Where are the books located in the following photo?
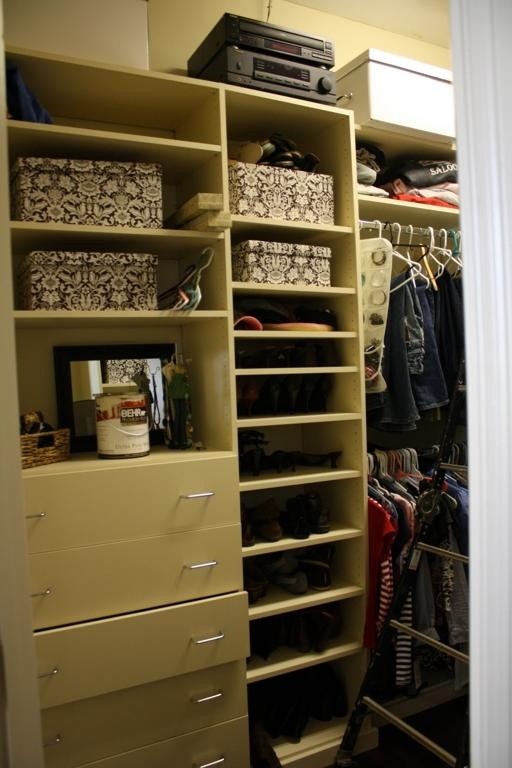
[14,249,159,312]
[229,158,335,225]
[335,47,455,138]
[231,238,332,289]
[10,157,164,230]
[2,0,148,71]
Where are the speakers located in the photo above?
[280,606,342,653]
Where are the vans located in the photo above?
[240,448,342,477]
[238,375,331,417]
[237,341,342,367]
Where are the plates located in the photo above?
[0,45,459,767]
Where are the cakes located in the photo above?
[95,392,150,459]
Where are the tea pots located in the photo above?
[52,344,178,455]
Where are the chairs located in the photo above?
[259,670,348,740]
[229,133,320,172]
[244,545,335,602]
[242,492,330,546]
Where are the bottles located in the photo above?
[234,310,263,330]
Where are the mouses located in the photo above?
[373,221,462,292]
[369,440,467,499]
[159,247,216,310]
